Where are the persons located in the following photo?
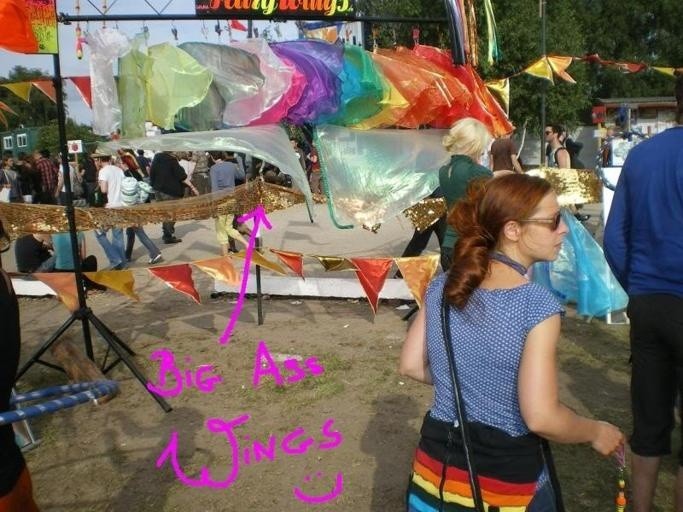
[602,78,680,512]
[1,269,42,512]
[0,117,588,291]
[397,173,627,511]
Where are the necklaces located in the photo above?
[489,253,526,277]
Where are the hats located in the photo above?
[89,148,111,158]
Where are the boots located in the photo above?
[162,227,182,244]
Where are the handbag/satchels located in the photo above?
[136,181,156,204]
[121,176,137,206]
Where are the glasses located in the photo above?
[516,211,560,230]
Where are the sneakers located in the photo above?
[148,252,162,264]
[109,262,131,270]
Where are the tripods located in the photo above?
[12,54,174,413]
